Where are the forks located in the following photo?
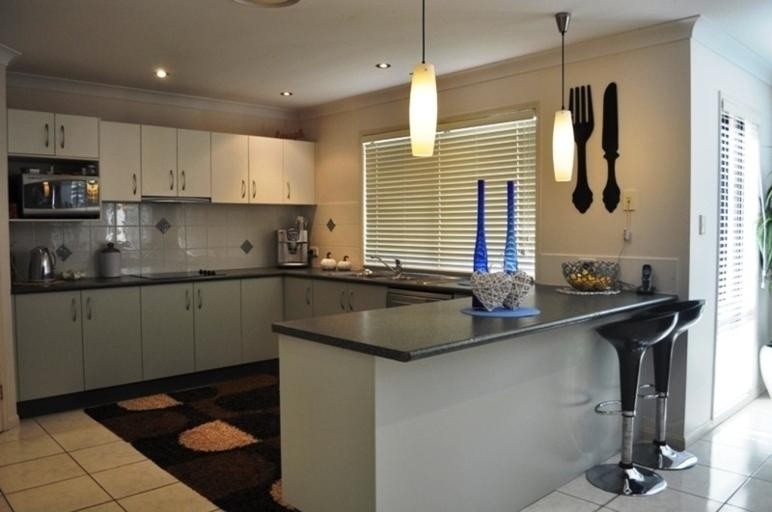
[566,85,594,215]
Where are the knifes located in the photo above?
[598,82,621,211]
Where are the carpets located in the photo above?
[84,359,302,511]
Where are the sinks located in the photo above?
[348,267,448,282]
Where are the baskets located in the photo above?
[561,260,621,292]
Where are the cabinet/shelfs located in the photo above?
[139,276,246,383]
[283,272,313,325]
[11,284,144,405]
[98,120,142,203]
[282,137,318,206]
[311,277,388,319]
[140,123,211,204]
[210,130,283,206]
[238,275,286,367]
[7,108,100,162]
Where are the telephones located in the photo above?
[636,264,655,295]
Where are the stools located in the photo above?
[585,307,682,498]
[632,298,707,473]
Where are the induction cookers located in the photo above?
[131,268,226,281]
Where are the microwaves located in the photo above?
[21,176,101,218]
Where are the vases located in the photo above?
[501,180,520,310]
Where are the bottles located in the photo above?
[99,242,122,279]
[502,181,518,275]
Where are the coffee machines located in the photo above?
[276,229,309,267]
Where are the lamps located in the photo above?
[407,0,438,159]
[550,11,576,184]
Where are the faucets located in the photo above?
[365,254,405,275]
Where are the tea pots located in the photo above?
[28,245,58,280]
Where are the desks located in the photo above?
[267,280,680,512]
